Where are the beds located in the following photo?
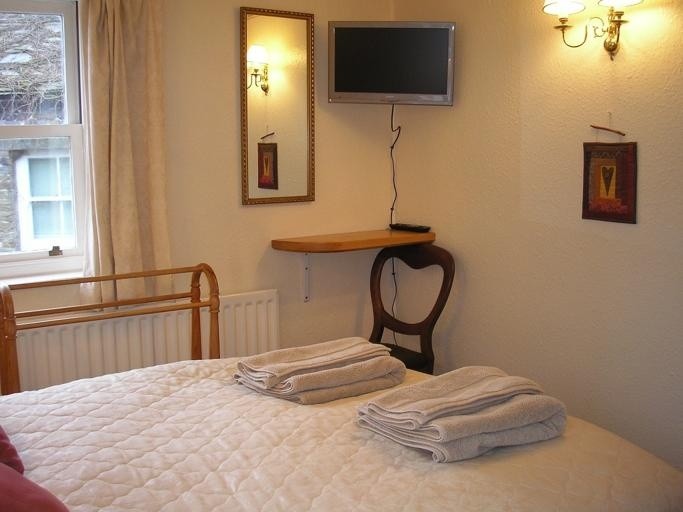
[1,357,683,511]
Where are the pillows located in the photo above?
[1,462,71,511]
[0,426,25,473]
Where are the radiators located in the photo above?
[16,289,278,391]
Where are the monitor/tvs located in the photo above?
[327,20,455,106]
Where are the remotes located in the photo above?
[390,223,430,233]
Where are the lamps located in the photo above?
[247,43,271,95]
[542,0,642,60]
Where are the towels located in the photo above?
[232,338,407,405]
[357,365,569,461]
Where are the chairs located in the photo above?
[368,242,455,374]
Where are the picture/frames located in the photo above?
[581,140,637,224]
[257,142,278,189]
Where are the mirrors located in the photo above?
[239,6,316,205]
